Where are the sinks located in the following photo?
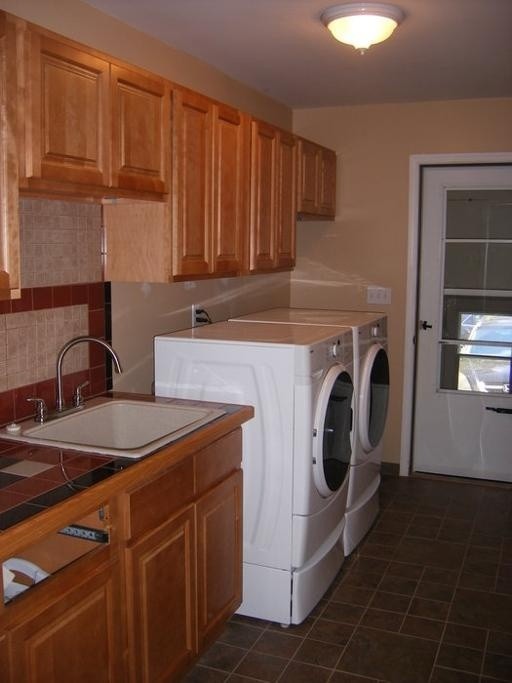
[2,396,228,460]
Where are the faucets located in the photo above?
[54,335,122,411]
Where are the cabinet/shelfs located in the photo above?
[0,424,243,683]
[21,19,173,202]
[101,81,296,284]
[295,133,337,218]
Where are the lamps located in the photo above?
[318,1,405,52]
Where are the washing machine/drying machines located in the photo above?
[231,305,391,558]
[153,321,358,626]
